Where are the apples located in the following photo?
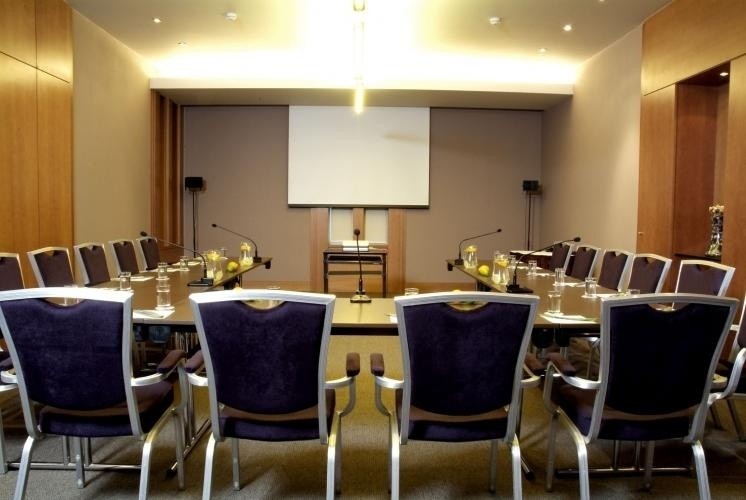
[479,264,490,276]
[227,261,238,273]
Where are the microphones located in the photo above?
[350,228,372,304]
[138,230,214,287]
[505,237,583,293]
[454,228,503,263]
[211,222,262,262]
[184,176,203,190]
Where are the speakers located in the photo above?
[523,180,538,190]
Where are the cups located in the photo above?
[405,288,420,295]
[64,241,282,308]
[463,243,641,314]
